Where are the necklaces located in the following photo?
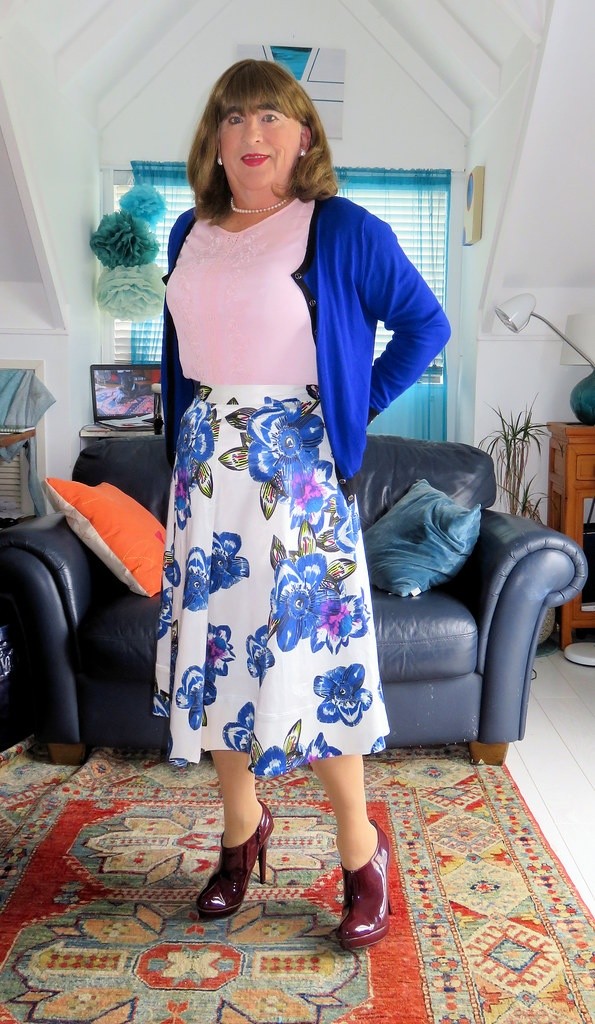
[230,197,287,213]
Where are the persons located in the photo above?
[160,58,455,950]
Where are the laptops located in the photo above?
[89,364,162,431]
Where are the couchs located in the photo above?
[5,432,590,767]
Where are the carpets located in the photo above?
[0,734,594,1024]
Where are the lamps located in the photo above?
[494,292,594,426]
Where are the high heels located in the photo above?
[335,821,393,950]
[196,800,274,918]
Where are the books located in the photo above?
[1,426,36,433]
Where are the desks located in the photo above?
[79,423,166,442]
[545,421,595,651]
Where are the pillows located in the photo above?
[362,479,482,598]
[45,476,166,598]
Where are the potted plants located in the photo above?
[476,392,555,648]
